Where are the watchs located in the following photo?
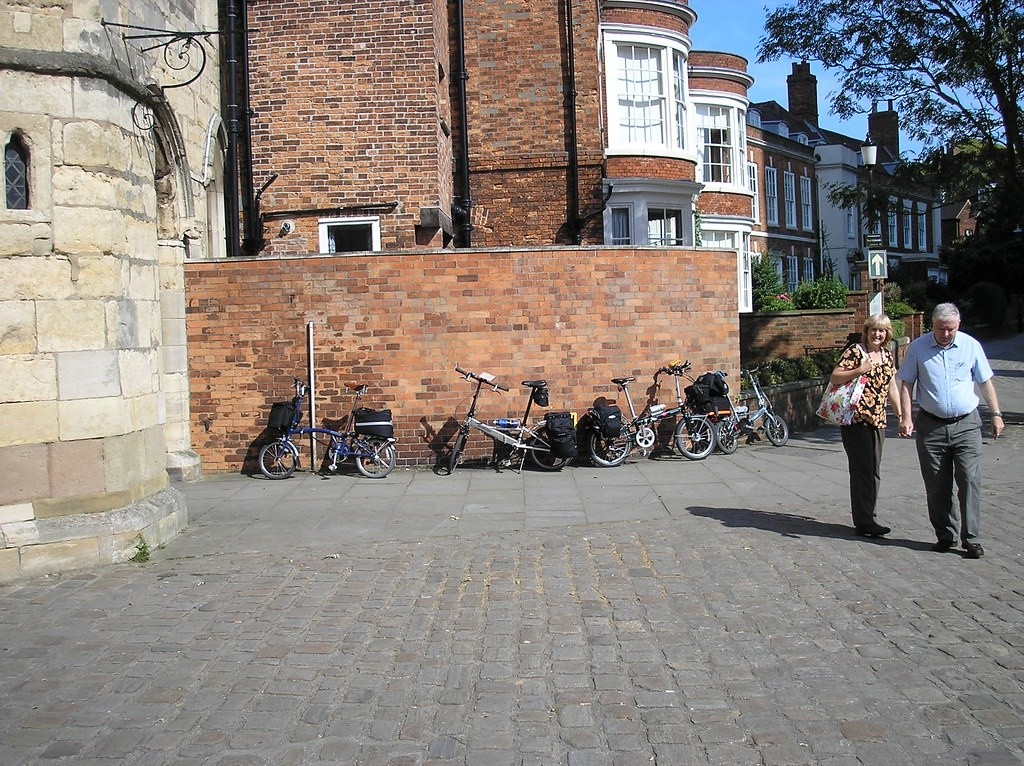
[990,412,1002,417]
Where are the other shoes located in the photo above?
[933,541,958,551]
[963,542,984,557]
[856,522,890,535]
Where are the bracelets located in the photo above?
[896,414,901,418]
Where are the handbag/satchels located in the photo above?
[816,343,868,425]
[693,372,729,395]
[545,417,578,458]
[595,406,621,438]
[268,401,303,429]
[698,397,730,412]
[352,407,394,437]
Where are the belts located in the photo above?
[920,408,968,424]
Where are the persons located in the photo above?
[898,302,1005,557]
[830,314,904,536]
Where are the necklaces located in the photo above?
[866,345,882,367]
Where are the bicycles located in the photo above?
[586,359,719,468]
[713,359,790,454]
[448,368,578,475]
[258,376,397,479]
